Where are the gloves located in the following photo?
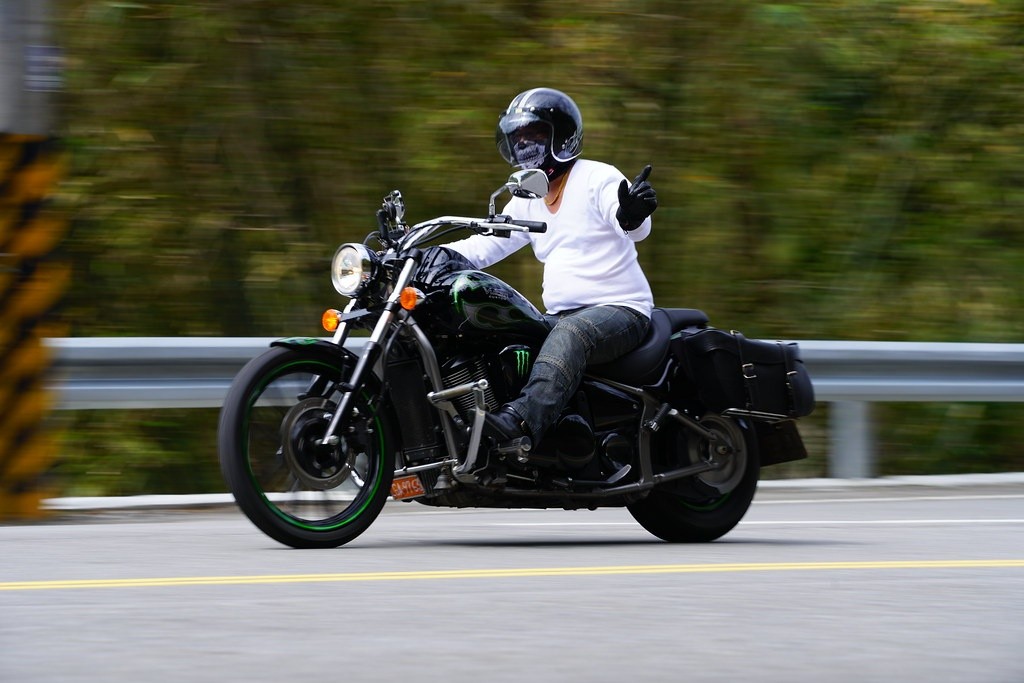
[616,163,659,231]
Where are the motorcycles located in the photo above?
[218,189,816,549]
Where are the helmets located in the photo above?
[494,87,585,165]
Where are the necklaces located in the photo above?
[544,172,568,206]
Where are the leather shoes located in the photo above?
[467,406,537,455]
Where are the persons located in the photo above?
[371,87,657,452]
